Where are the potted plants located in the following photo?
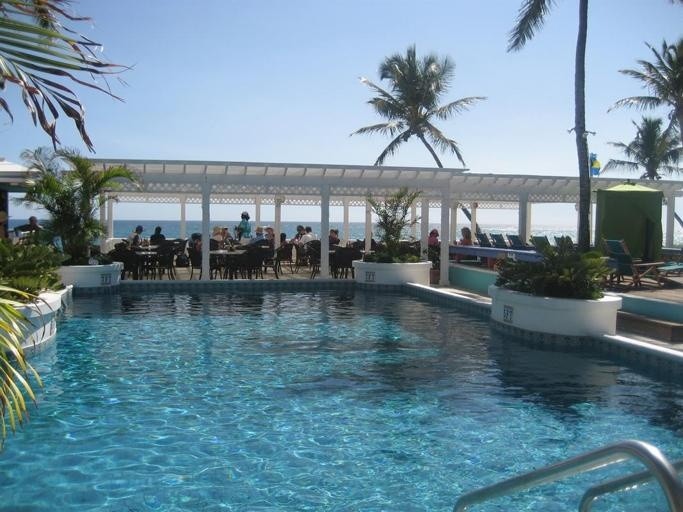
[487,233,622,337]
[0,230,72,354]
[10,145,146,289]
[351,184,433,290]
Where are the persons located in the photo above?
[427,228,438,246]
[20,216,43,231]
[123,224,146,265]
[361,230,378,249]
[148,226,165,253]
[190,210,341,264]
[453,226,471,260]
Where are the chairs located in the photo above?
[474,232,664,294]
[107,239,361,280]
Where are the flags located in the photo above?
[589,153,601,176]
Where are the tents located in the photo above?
[594,179,664,261]
